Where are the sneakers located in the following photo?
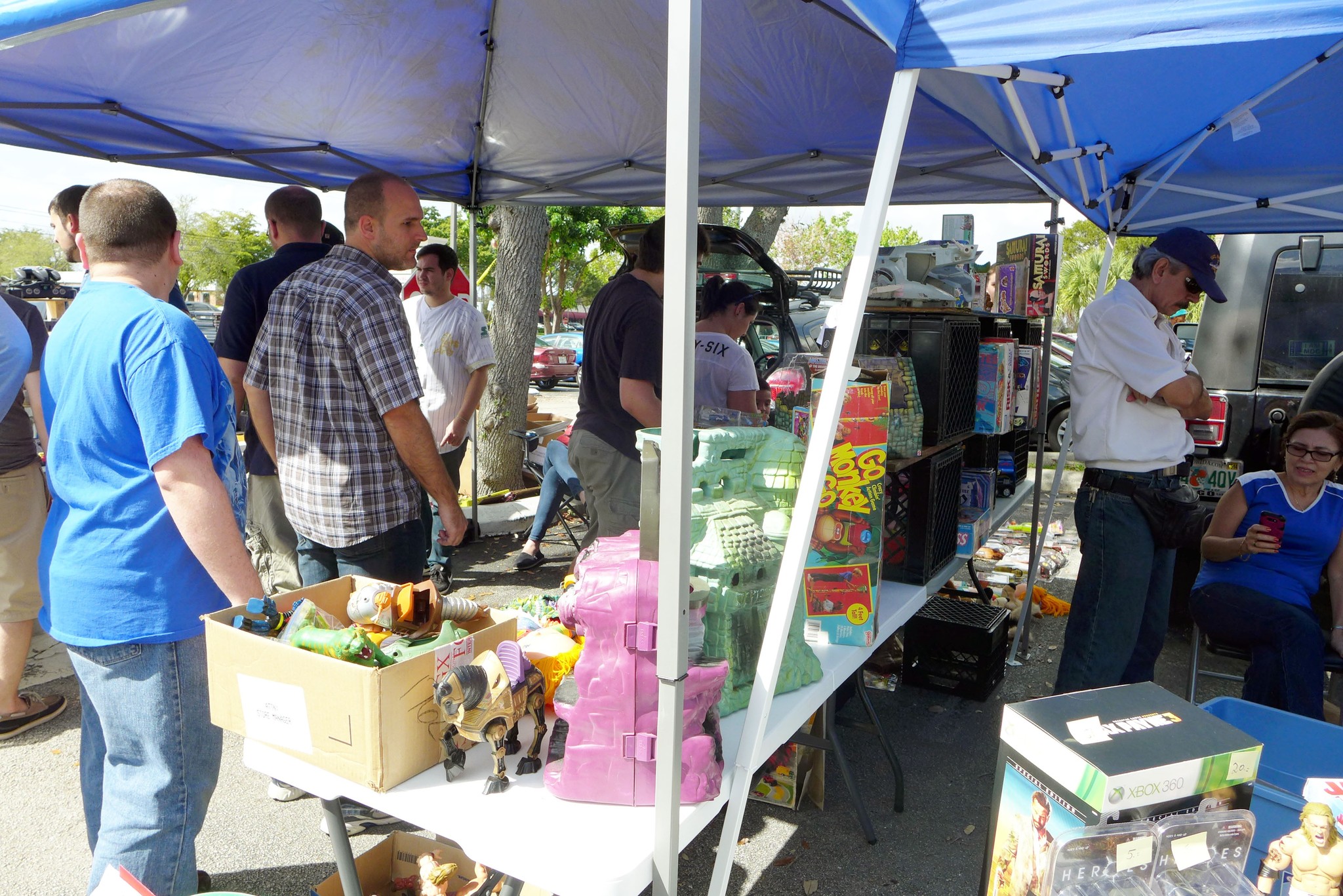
[422,565,430,576]
[429,564,453,595]
[267,777,307,801]
[0,691,67,741]
[319,808,403,836]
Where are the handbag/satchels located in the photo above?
[1082,468,1206,549]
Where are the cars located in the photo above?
[531,330,586,390]
[185,302,222,324]
[537,321,586,332]
[1024,330,1079,449]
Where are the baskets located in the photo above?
[979,317,1011,338]
[900,595,1011,702]
[1000,429,1031,486]
[964,433,999,510]
[856,312,981,446]
[1011,322,1042,345]
[882,442,964,586]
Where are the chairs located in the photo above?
[1184,511,1343,723]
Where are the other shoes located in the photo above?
[512,550,544,570]
[197,870,211,894]
[584,500,590,517]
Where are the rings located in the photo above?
[1254,541,1257,547]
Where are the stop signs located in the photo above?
[563,318,569,324]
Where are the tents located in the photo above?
[0,2,1062,895]
[702,2,1343,896]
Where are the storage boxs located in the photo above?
[746,700,828,815]
[900,595,1012,701]
[524,412,575,469]
[197,572,516,794]
[775,231,1064,647]
[976,680,1265,894]
[1192,694,1343,895]
[307,827,557,895]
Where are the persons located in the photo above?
[512,440,589,570]
[1,295,35,424]
[36,178,267,896]
[691,275,761,429]
[401,243,496,595]
[0,289,69,743]
[48,185,190,317]
[1052,226,1228,695]
[1255,801,1343,895]
[242,170,471,837]
[1192,411,1343,720]
[992,790,1055,896]
[565,214,710,551]
[211,186,335,802]
[755,375,772,426]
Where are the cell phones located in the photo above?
[1256,510,1285,547]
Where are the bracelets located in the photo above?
[456,416,468,424]
[1331,626,1343,630]
[1237,537,1251,561]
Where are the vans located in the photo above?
[1169,226,1343,621]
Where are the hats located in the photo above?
[1149,226,1228,303]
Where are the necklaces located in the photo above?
[1289,482,1319,511]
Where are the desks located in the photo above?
[235,475,1054,894]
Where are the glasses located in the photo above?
[1286,440,1343,462]
[266,219,275,236]
[1185,277,1202,294]
